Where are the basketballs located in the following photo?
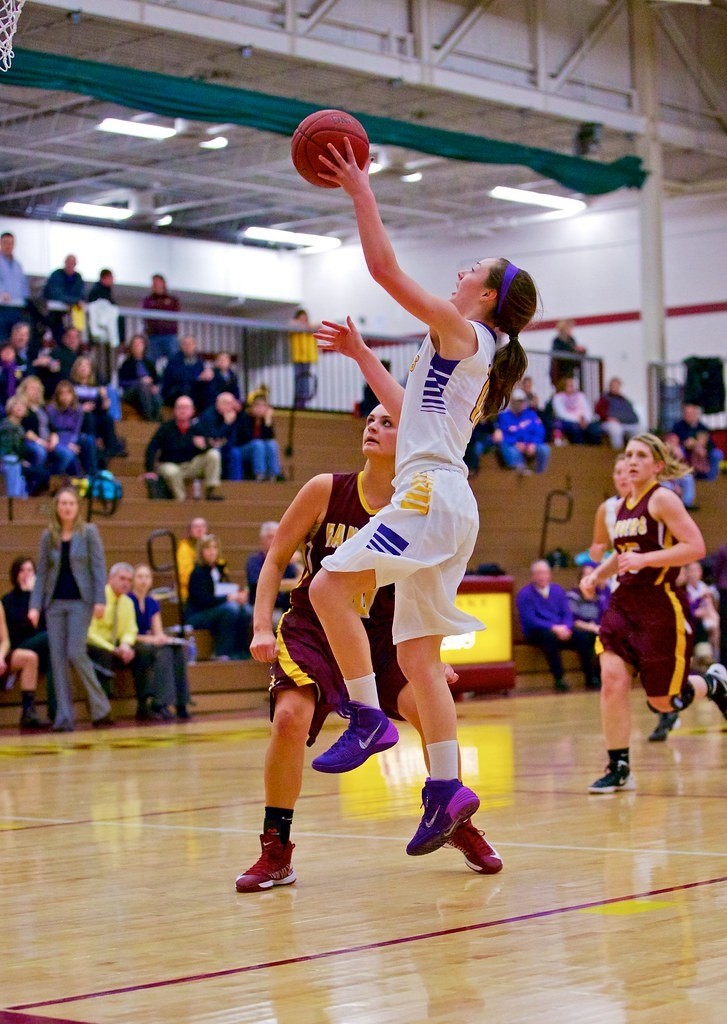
[291,109,371,190]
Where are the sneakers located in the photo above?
[646,713,680,744]
[236,835,296,892]
[706,663,727,721]
[588,754,637,793]
[312,688,399,773]
[407,778,480,856]
[442,819,503,874]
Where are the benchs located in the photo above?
[0,409,727,705]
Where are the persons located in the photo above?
[0,233,727,733]
[312,137,537,854]
[582,433,727,792]
[590,453,693,742]
[235,404,502,895]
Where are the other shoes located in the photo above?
[21,715,49,728]
[136,706,173,721]
[173,707,191,722]
[206,491,225,502]
[93,713,114,728]
[586,673,602,691]
[685,503,702,512]
[553,676,570,694]
[210,652,235,666]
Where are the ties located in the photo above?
[112,600,117,648]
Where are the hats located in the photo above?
[512,389,528,402]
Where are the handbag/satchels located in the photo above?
[84,470,123,522]
